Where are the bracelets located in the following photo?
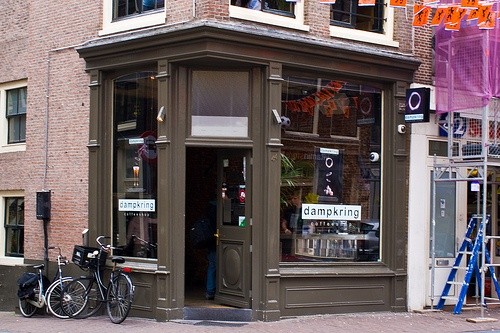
[285,229,288,232]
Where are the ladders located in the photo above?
[437,214,500,314]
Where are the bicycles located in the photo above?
[17,246,89,320]
[59,236,136,324]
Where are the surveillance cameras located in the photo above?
[157,107,166,122]
[272,109,282,125]
[398,125,406,134]
[369,152,379,162]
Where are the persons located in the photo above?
[282,192,315,254]
[127,215,157,257]
[202,192,218,300]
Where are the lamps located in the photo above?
[156,106,166,121]
[272,109,282,125]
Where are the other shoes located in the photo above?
[208,294,214,300]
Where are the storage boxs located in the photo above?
[72,246,108,268]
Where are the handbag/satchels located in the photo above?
[17,272,39,301]
